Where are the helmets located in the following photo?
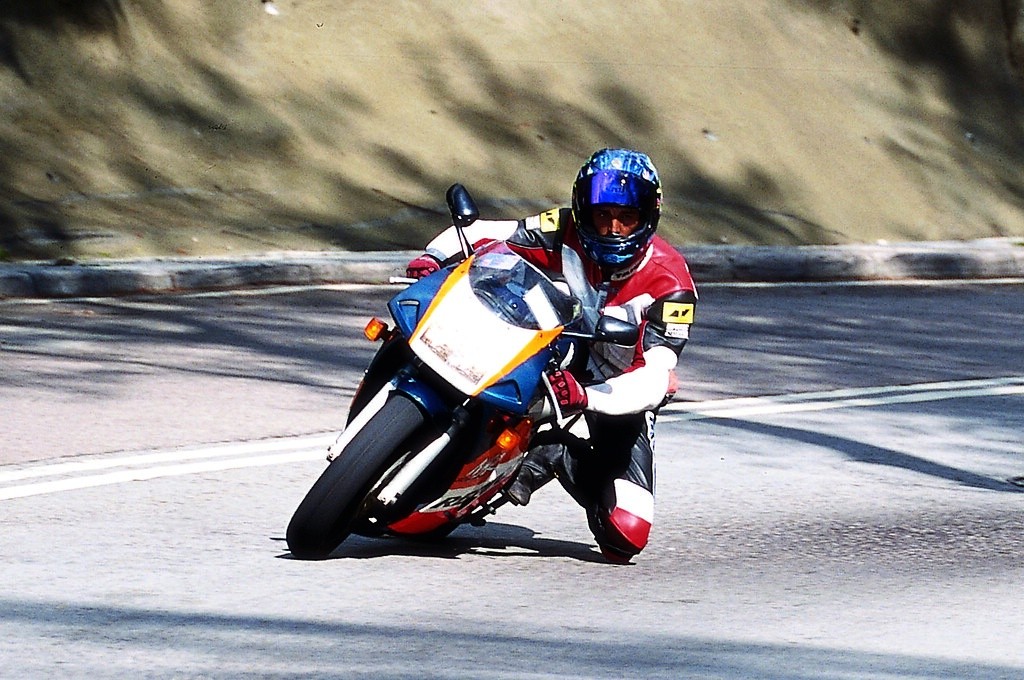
[571,146,663,268]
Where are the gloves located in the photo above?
[550,368,588,412]
[406,253,442,283]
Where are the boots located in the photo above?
[506,430,598,512]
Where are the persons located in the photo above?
[407,149,699,564]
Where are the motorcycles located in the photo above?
[286,182,681,561]
